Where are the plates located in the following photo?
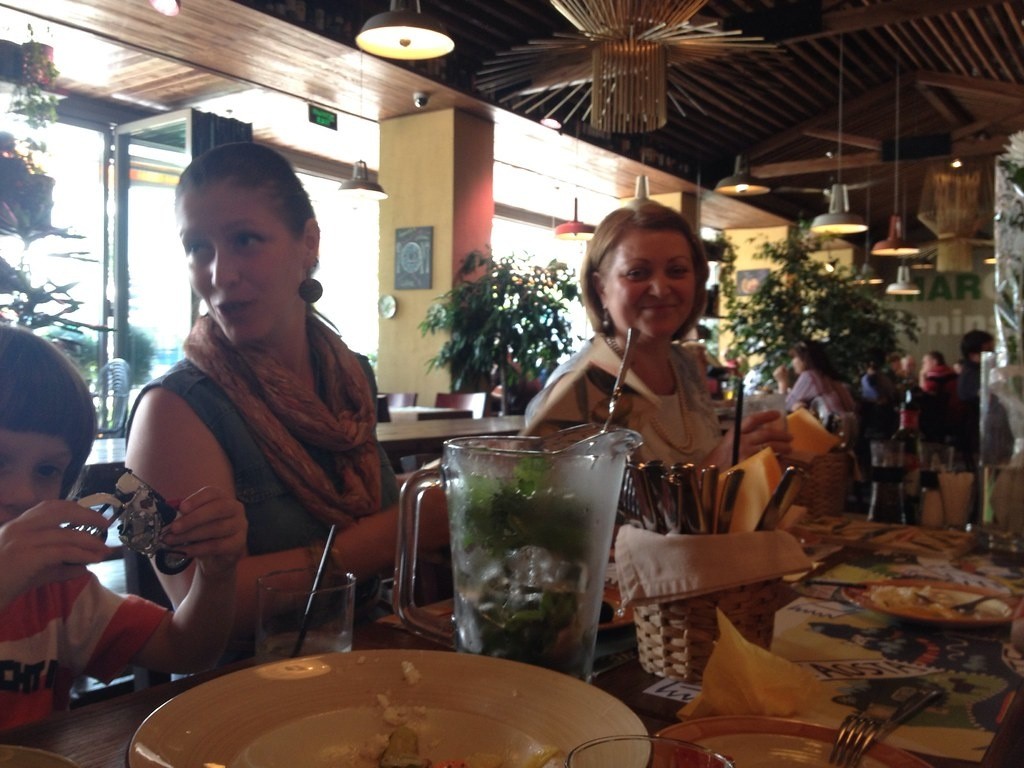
[842,579,1023,627]
[596,583,639,628]
[647,716,934,768]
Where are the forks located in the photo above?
[828,688,943,766]
[619,470,644,520]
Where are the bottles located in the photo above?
[888,386,923,524]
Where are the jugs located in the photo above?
[391,421,643,682]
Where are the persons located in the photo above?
[773,341,854,425]
[124,141,532,683]
[507,197,793,528]
[862,331,1015,476]
[0,326,248,728]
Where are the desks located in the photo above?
[375,416,526,475]
[388,406,475,473]
[80,437,126,466]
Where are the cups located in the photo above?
[867,437,906,523]
[564,735,733,768]
[980,350,1024,551]
[256,568,356,662]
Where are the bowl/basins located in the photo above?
[0,743,77,768]
[126,650,651,768]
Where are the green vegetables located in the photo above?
[452,451,590,670]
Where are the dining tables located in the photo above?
[0,503,1024,768]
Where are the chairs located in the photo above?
[61,462,134,563]
[433,392,487,420]
[376,395,404,474]
[378,392,418,407]
[92,359,133,439]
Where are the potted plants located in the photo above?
[0,20,61,227]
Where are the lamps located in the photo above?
[825,258,839,273]
[354,0,455,60]
[623,148,662,210]
[951,157,963,168]
[984,255,996,264]
[884,159,920,295]
[870,40,920,256]
[553,92,596,240]
[852,167,887,285]
[333,50,389,201]
[909,256,934,270]
[539,108,562,129]
[713,56,772,196]
[148,0,180,17]
[806,33,870,234]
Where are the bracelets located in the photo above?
[313,542,338,621]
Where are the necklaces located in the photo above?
[606,337,696,453]
